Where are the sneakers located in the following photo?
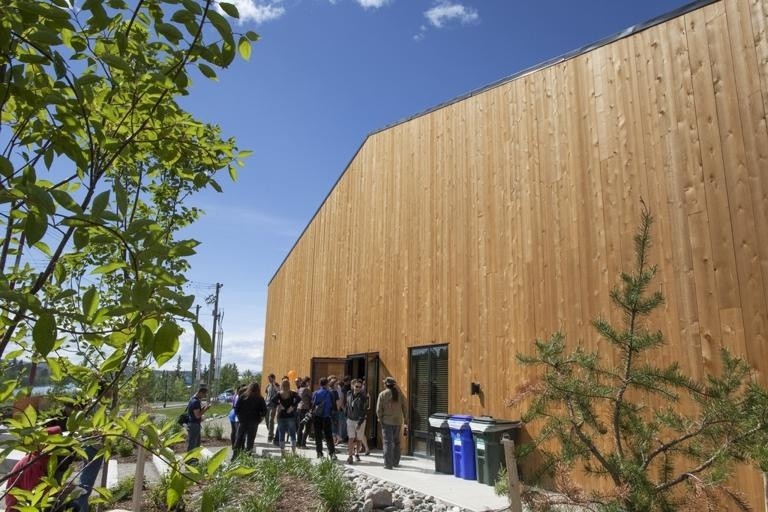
[265,438,399,470]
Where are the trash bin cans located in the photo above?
[428,412,521,486]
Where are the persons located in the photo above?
[4,380,108,512]
[185,388,212,465]
[227,373,371,464]
[375,377,409,470]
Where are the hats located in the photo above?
[382,376,397,386]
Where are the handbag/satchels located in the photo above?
[315,400,324,417]
[177,413,189,424]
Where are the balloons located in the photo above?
[288,369,296,380]
